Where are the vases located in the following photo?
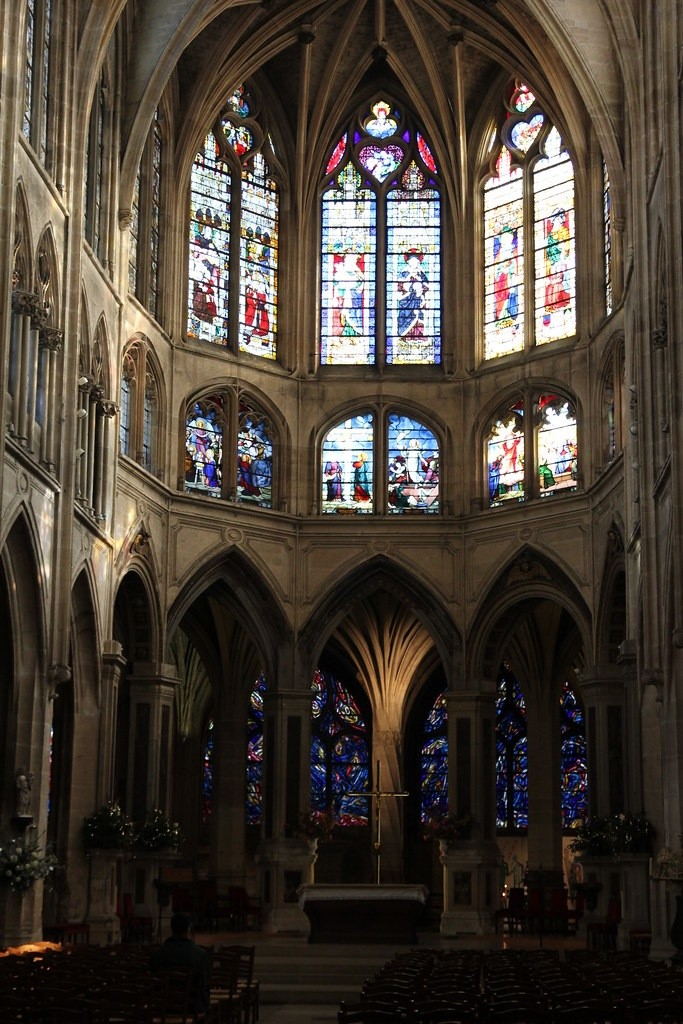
[439,838,449,856]
[308,838,320,855]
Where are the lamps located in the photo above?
[628,384,636,393]
[632,462,639,471]
[75,448,85,458]
[630,425,638,435]
[77,408,89,419]
[78,376,89,386]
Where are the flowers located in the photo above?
[300,811,334,846]
[418,815,460,841]
[0,833,60,898]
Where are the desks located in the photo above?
[43,922,90,943]
[298,883,431,945]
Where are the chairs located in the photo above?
[117,893,153,942]
[338,949,683,1024]
[172,880,263,933]
[0,943,262,1024]
[494,880,623,949]
[628,929,652,953]
